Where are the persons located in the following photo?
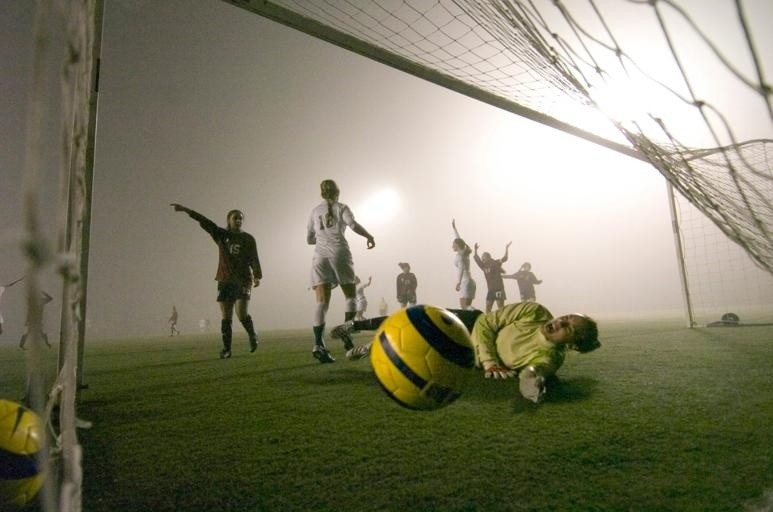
[329,301,602,405]
[395,263,419,310]
[451,217,477,311]
[473,238,513,316]
[379,297,390,317]
[20,286,54,351]
[306,179,378,365]
[501,261,544,303]
[167,306,182,339]
[1,274,28,337]
[168,202,264,360]
[353,275,373,323]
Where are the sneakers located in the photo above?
[346,343,372,361]
[220,349,231,359]
[248,333,258,352]
[312,345,336,362]
[341,332,353,351]
[329,320,360,339]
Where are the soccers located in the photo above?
[370,304,476,412]
[0,400,49,511]
